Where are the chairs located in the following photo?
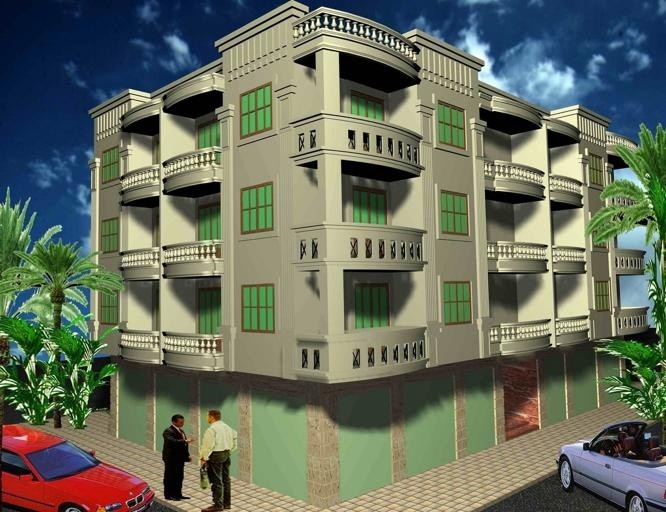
[622,436,662,461]
